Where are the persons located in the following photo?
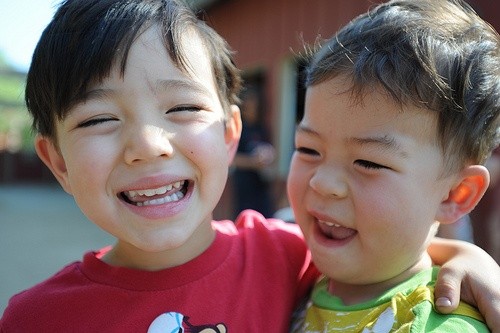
[227,94,277,220]
[285,0,500,333]
[0,1,500,333]
